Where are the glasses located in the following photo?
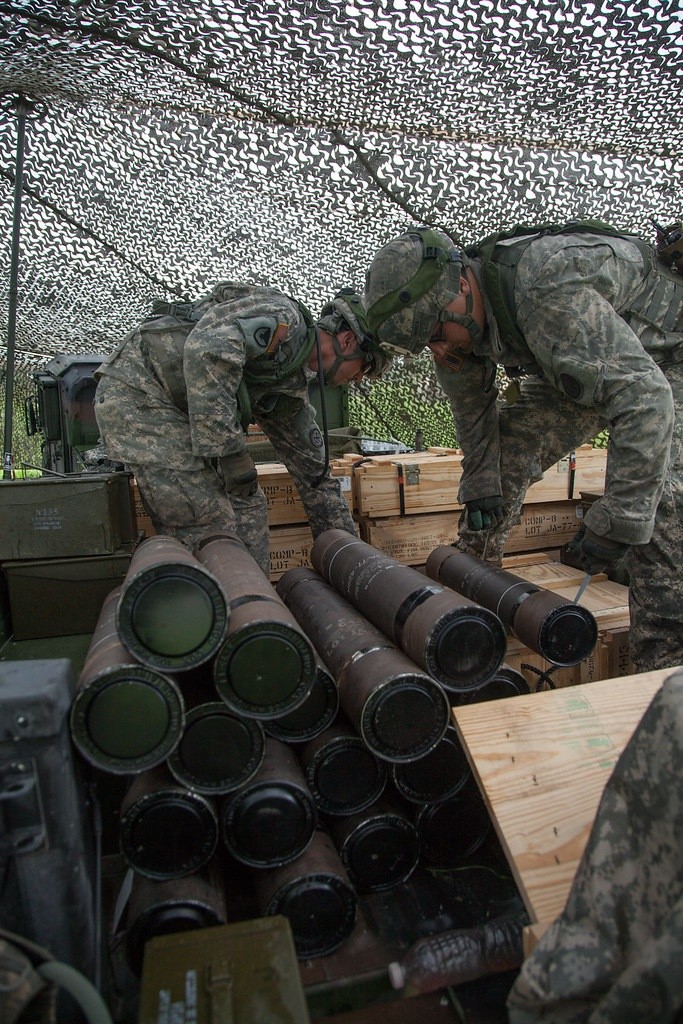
[343,324,375,374]
[429,322,447,343]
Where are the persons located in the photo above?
[366,220,683,673]
[506,668,683,1024]
[93,281,392,579]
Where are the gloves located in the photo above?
[220,448,258,498]
[569,523,633,575]
[466,496,506,531]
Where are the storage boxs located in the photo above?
[2,530,147,641]
[135,442,632,693]
[0,471,139,555]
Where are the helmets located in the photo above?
[321,288,395,380]
[363,227,462,358]
